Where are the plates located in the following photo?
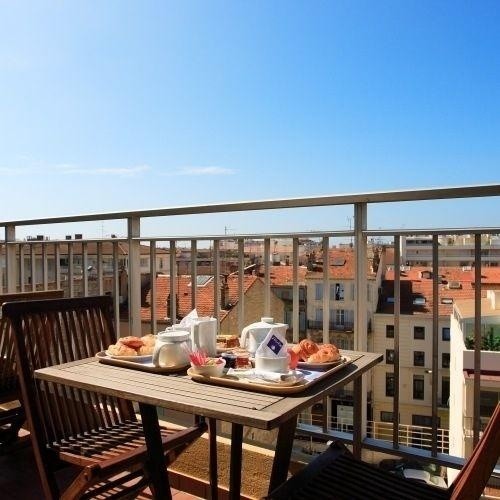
[217,348,236,353]
[105,350,153,362]
[242,355,346,386]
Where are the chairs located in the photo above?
[0,290,65,446]
[0,294,208,500]
[259,400,500,500]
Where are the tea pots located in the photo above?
[240,317,291,367]
[152,330,191,368]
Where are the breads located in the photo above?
[293,339,340,363]
[108,334,157,356]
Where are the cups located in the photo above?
[222,353,250,370]
[190,358,226,377]
[191,318,217,356]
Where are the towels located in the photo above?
[226,366,305,386]
